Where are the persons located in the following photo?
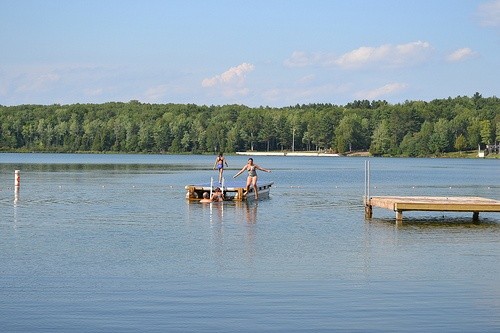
[211,187,224,201]
[233,157,271,200]
[199,192,212,202]
[213,152,228,184]
